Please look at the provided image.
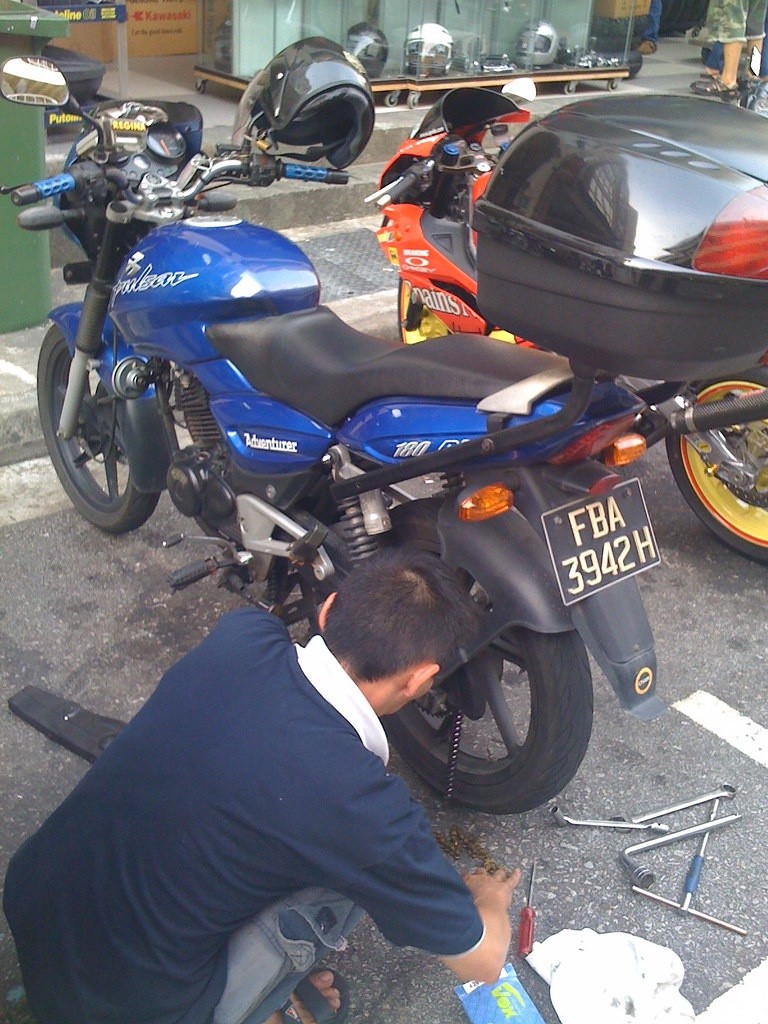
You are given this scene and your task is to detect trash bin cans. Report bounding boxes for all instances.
[0,0,74,338]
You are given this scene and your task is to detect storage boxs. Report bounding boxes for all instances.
[477,92,768,381]
[46,0,231,65]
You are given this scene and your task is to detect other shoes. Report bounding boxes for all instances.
[638,40,657,54]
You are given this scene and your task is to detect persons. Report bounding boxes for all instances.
[3,545,521,1024]
[689,0,768,100]
[635,0,662,55]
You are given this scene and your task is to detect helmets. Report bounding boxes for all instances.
[345,22,388,77]
[213,18,234,72]
[404,23,453,77]
[231,36,375,170]
[515,19,559,66]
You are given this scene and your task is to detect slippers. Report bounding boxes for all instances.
[700,72,721,80]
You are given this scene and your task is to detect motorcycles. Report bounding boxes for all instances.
[0,54,677,815]
[363,75,767,565]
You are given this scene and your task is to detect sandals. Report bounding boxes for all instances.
[689,77,738,104]
[736,77,748,90]
[279,968,348,1024]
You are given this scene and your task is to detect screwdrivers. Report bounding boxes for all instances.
[519,859,540,954]
